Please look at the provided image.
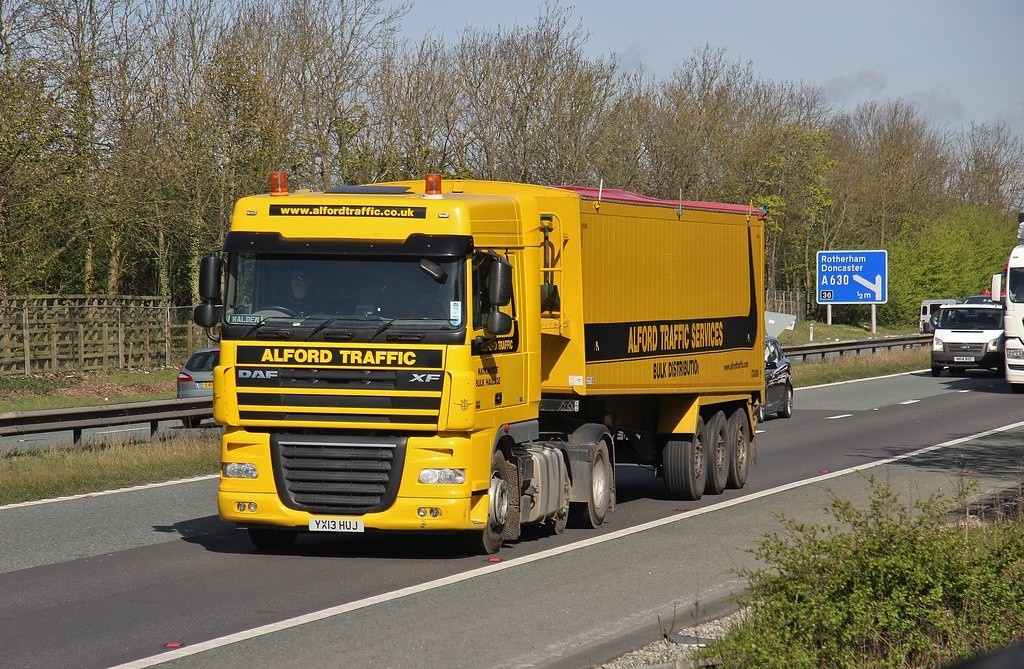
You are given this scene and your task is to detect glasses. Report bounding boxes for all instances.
[289,274,309,281]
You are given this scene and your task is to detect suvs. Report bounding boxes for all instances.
[919,300,962,334]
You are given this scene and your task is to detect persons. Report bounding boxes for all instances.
[375,268,447,318]
[270,266,326,317]
[978,313,987,320]
[953,311,961,323]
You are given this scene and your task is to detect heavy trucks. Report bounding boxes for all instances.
[193,172,766,555]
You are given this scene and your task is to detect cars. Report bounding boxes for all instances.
[754,336,793,423]
[177,348,220,427]
[931,304,1005,377]
[949,296,1005,320]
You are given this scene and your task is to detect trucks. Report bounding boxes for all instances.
[991,213,1024,393]
